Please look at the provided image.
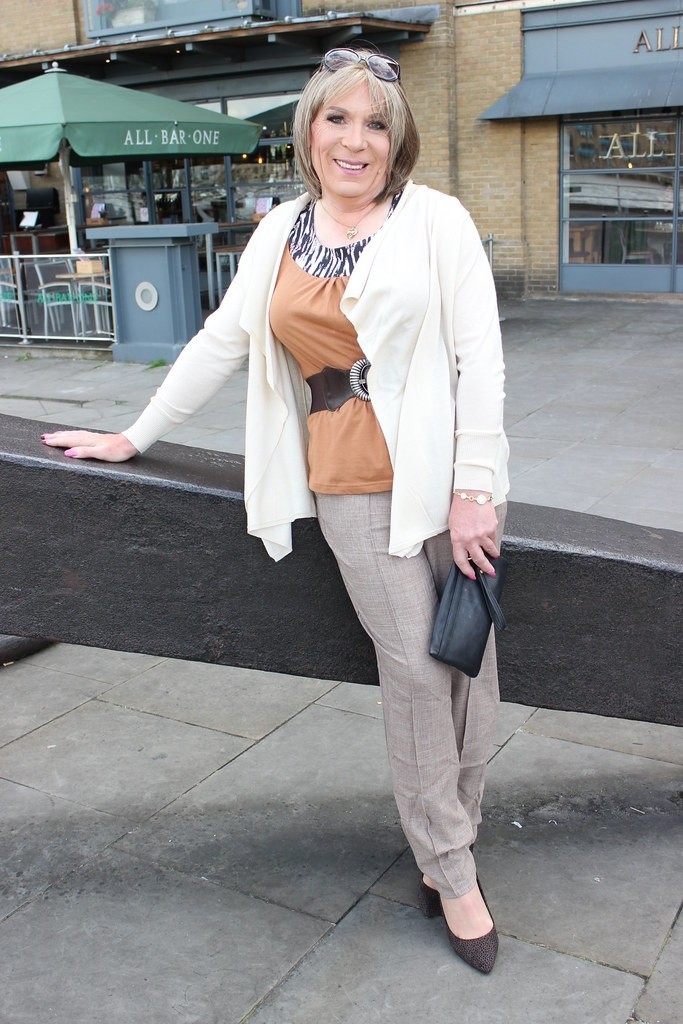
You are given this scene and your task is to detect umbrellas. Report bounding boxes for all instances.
[0,62,266,254]
[243,100,306,136]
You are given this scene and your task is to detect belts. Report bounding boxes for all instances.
[305,360,372,415]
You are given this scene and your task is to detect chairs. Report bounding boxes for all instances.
[618,228,655,264]
[1,256,115,343]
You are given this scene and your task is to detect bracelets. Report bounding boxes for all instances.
[450,490,494,504]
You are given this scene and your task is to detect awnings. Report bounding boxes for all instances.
[569,173,683,213]
[475,61,683,120]
[0,5,440,87]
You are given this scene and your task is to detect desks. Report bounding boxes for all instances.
[206,220,260,310]
[635,227,683,265]
[55,272,115,337]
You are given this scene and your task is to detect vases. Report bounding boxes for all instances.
[110,7,155,28]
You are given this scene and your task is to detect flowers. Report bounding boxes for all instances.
[96,0,158,20]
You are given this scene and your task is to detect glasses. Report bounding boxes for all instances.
[319,48,402,85]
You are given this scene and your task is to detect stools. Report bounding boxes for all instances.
[215,245,247,308]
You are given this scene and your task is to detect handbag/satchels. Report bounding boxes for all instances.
[429,542,509,678]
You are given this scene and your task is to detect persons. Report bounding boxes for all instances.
[40,48,510,976]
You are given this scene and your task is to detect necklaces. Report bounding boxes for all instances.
[317,197,380,238]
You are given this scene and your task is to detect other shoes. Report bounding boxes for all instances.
[440,866,498,974]
[416,841,474,918]
